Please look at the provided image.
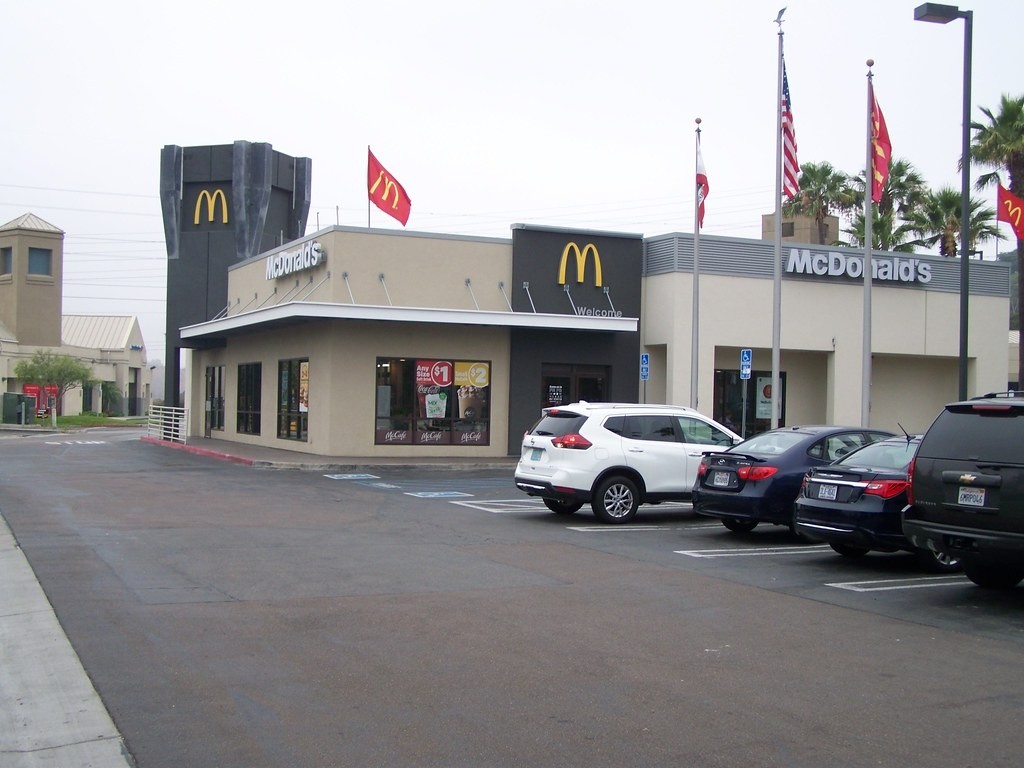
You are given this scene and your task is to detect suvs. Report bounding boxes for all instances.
[900,390,1024,592]
[514,400,782,523]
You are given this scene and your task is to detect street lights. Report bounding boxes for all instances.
[914,2,973,401]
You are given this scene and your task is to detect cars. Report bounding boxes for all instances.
[690,423,898,544]
[393,412,487,433]
[792,435,964,575]
